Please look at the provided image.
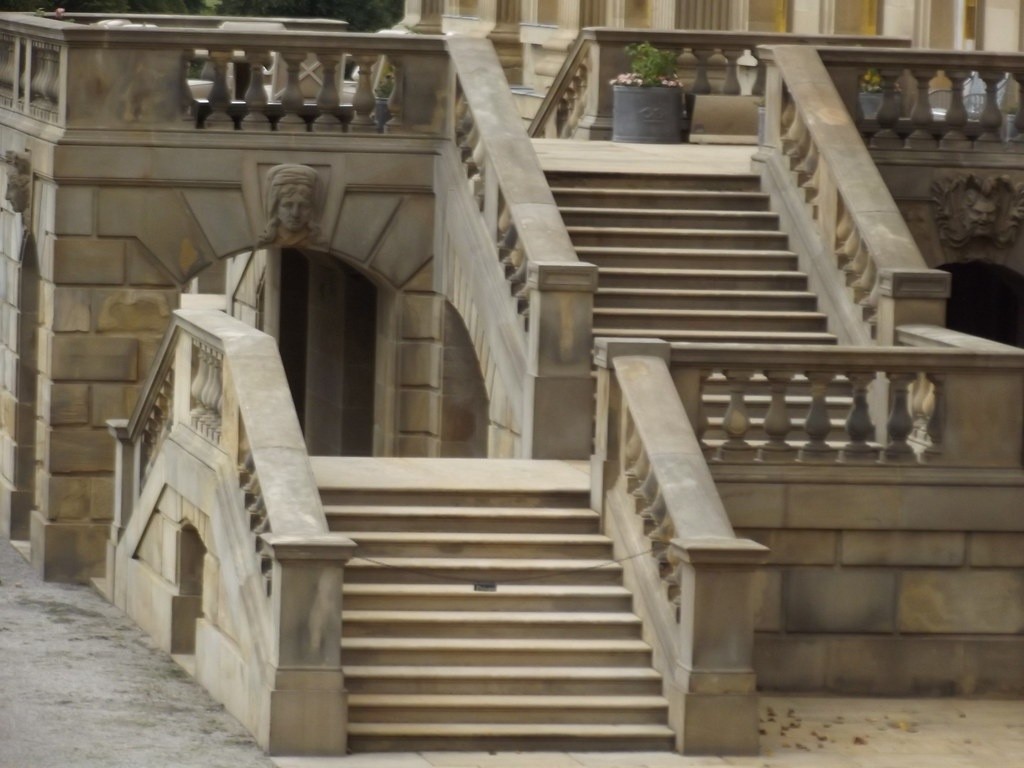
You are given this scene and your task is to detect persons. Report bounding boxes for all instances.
[925,171,1023,259]
[259,165,325,246]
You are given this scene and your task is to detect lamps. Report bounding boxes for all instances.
[331,104,357,132]
[296,104,323,132]
[191,98,214,129]
[260,102,287,132]
[226,100,250,130]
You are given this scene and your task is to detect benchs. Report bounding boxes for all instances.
[928,70,1010,123]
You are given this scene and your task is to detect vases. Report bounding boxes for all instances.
[859,93,904,121]
[610,84,684,145]
[373,98,395,133]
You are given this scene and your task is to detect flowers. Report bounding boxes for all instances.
[607,40,686,89]
[375,64,398,98]
[860,68,904,94]
